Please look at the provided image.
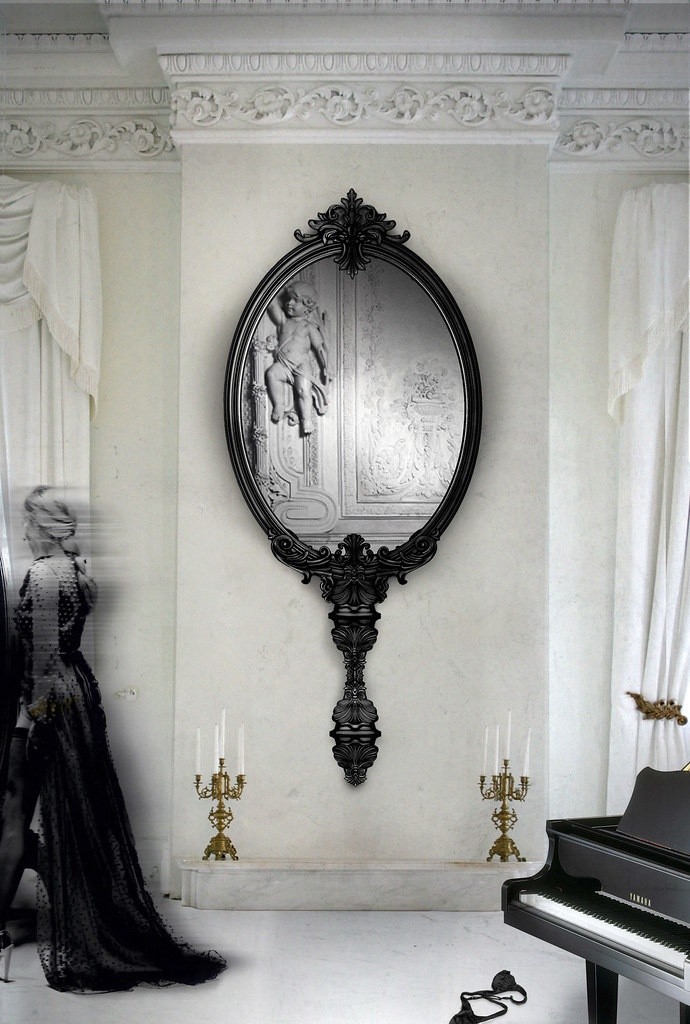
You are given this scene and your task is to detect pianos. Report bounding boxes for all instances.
[500,762,690,1024]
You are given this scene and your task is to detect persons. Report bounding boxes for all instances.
[264,279,333,434]
[1,485,229,994]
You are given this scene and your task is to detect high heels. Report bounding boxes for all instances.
[0,931,13,982]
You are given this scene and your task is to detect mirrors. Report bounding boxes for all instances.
[224,187,483,790]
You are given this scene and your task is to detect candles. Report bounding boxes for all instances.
[494,725,498,775]
[219,708,225,758]
[237,726,245,775]
[504,709,512,759]
[522,727,531,776]
[480,726,488,776]
[195,726,200,774]
[213,722,218,775]
